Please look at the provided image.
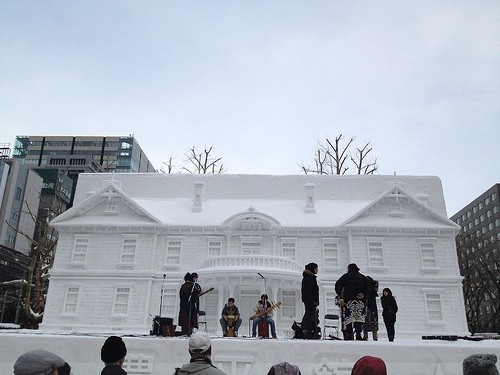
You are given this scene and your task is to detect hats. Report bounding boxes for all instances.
[351,356,387,375]
[101,336,126,363]
[267,362,301,375]
[463,354,499,375]
[189,332,211,354]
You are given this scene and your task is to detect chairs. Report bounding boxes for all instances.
[322,314,340,340]
[198,311,207,332]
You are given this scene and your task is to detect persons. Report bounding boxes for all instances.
[13,349,65,375]
[101,336,127,375]
[301,262,319,338]
[463,353,499,375]
[362,275,379,341]
[252,294,277,339]
[177,332,227,375]
[335,264,368,340]
[351,356,387,375]
[267,362,301,375]
[380,288,398,342]
[220,297,242,337]
[178,272,202,337]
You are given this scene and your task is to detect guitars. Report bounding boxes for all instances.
[198,288,214,297]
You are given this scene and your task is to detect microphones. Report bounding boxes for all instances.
[163,274,166,278]
[258,272,264,279]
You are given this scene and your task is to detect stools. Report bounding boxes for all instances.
[258,322,270,339]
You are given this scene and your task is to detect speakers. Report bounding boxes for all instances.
[153,317,173,335]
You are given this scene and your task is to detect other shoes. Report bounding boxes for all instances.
[272,333,277,338]
[223,330,227,336]
[252,333,256,337]
[356,339,362,341]
[362,338,368,341]
[374,339,377,341]
[304,333,315,339]
[389,340,394,342]
[233,329,236,337]
[346,338,354,340]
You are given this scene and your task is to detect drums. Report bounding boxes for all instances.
[226,315,236,337]
